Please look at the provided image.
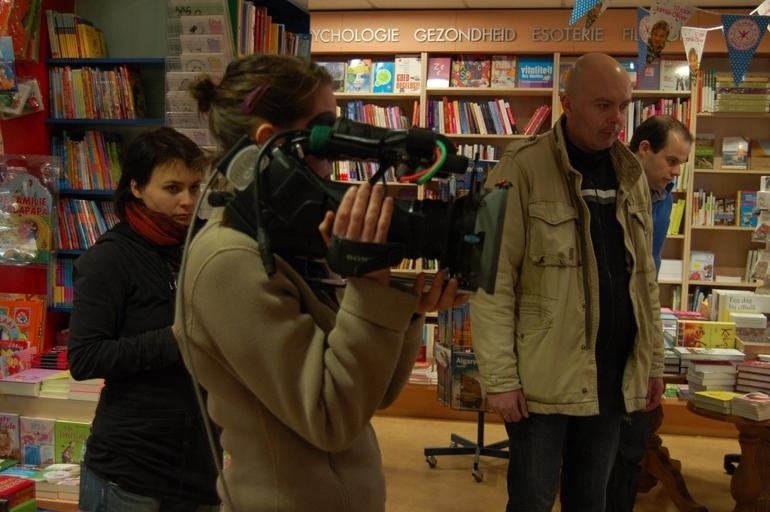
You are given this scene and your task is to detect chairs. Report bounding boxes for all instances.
[425,398,534,485]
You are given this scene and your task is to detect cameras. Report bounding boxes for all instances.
[206,116,514,297]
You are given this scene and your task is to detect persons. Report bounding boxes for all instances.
[172,54,469,511]
[468,54,666,511]
[68,126,221,512]
[606,114,694,510]
[386,257,442,271]
[649,18,670,51]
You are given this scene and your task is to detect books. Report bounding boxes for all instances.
[558,54,690,94]
[46,8,107,58]
[696,65,768,113]
[618,94,690,147]
[50,129,125,191]
[694,133,769,172]
[326,99,420,131]
[692,229,769,283]
[694,176,769,229]
[668,194,686,239]
[427,56,553,89]
[48,63,148,120]
[417,143,501,202]
[654,158,690,193]
[391,283,484,412]
[424,96,552,135]
[161,13,235,221]
[329,158,417,185]
[1,328,106,401]
[50,259,75,307]
[658,256,683,285]
[312,55,422,94]
[658,285,769,422]
[1,413,91,512]
[241,1,312,55]
[52,199,121,251]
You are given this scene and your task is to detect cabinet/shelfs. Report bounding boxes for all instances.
[312,9,770,440]
[47,58,165,376]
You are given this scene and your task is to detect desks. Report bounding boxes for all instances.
[686,401,769,512]
